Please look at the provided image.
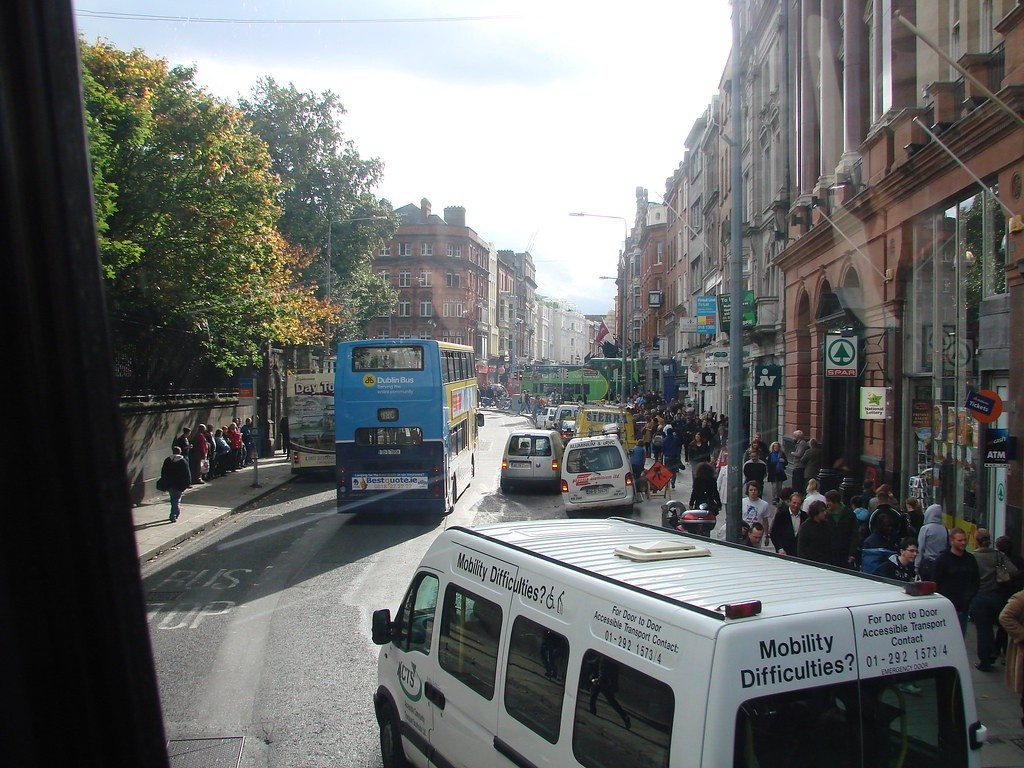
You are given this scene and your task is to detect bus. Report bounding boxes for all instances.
[333,339,485,522]
[286,366,336,474]
[520,356,647,408]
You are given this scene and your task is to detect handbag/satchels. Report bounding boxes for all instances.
[937,527,952,567]
[707,496,719,515]
[996,550,1010,581]
[642,427,646,435]
[905,513,916,536]
[775,452,784,473]
[156,477,167,491]
[200,458,209,473]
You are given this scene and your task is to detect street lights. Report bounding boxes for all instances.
[567,212,629,405]
[323,214,387,375]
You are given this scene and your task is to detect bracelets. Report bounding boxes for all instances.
[765,536,769,538]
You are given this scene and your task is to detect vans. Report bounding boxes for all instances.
[551,405,581,435]
[500,429,565,494]
[369,515,989,768]
[572,400,636,457]
[561,434,634,519]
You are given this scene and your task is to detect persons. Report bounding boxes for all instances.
[518,389,531,415]
[541,632,559,677]
[609,390,729,516]
[744,432,789,504]
[280,416,290,462]
[972,528,1019,670]
[771,460,981,692]
[477,385,501,406]
[999,590,1024,730]
[791,429,817,493]
[588,656,631,730]
[531,387,572,406]
[741,482,770,548]
[177,415,259,488]
[161,446,191,522]
[576,392,587,404]
[994,536,1024,664]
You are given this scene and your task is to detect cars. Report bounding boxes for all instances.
[561,419,577,447]
[534,407,558,430]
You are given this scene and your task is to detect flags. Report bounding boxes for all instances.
[596,321,609,342]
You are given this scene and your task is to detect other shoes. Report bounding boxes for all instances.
[990,657,995,663]
[975,663,991,671]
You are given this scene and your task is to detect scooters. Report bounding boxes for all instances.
[661,502,717,537]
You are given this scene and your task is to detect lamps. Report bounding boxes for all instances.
[904,144,925,155]
[963,96,986,110]
[790,214,816,226]
[812,196,823,207]
[931,122,952,135]
[775,231,796,241]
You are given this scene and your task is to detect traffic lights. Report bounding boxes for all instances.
[519,370,523,381]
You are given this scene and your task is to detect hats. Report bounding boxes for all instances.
[667,428,673,434]
[825,489,841,501]
